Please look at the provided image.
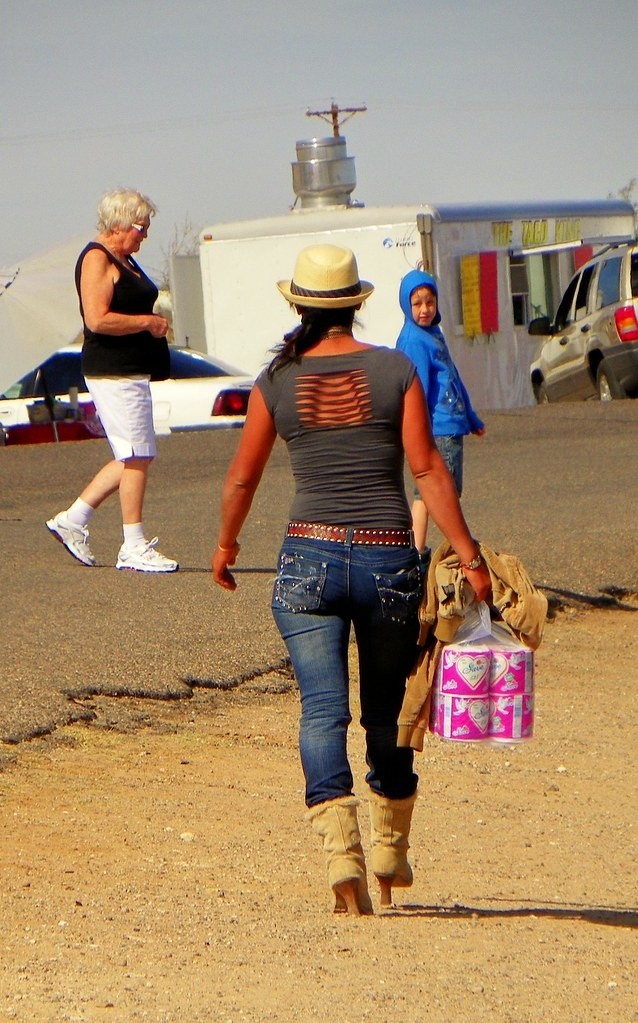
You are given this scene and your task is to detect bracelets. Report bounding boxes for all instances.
[463,556,481,569]
[218,542,238,552]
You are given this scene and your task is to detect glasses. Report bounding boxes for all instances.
[130,222,150,234]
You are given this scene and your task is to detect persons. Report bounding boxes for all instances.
[47,190,179,572]
[395,270,486,567]
[211,244,490,915]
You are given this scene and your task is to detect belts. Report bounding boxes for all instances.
[287,520,411,546]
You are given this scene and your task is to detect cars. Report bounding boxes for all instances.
[0,344,255,435]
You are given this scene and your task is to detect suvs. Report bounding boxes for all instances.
[527,240,638,403]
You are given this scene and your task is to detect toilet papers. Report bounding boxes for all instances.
[428,642,536,744]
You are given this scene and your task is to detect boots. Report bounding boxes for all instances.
[364,788,413,906]
[303,795,374,917]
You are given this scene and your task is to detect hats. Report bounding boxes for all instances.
[275,243,375,308]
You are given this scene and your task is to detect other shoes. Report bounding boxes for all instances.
[417,545,432,575]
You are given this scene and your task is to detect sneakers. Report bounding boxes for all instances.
[116,534,179,573]
[45,510,96,567]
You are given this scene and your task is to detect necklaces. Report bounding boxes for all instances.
[325,330,353,336]
